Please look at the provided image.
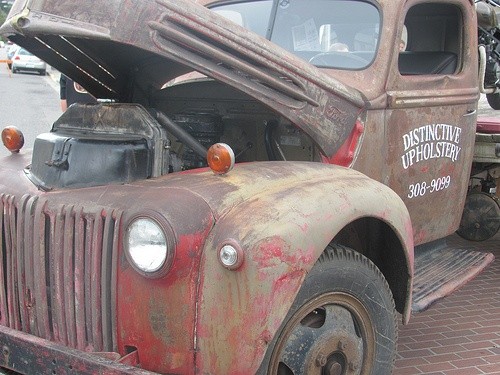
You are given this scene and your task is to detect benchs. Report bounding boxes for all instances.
[293,50,459,75]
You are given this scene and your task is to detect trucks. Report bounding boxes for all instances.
[0,0,500,375]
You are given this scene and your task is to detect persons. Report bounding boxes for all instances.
[60,74,95,113]
[330,43,348,51]
[400,40,405,51]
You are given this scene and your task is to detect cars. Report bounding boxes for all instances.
[8,44,46,75]
